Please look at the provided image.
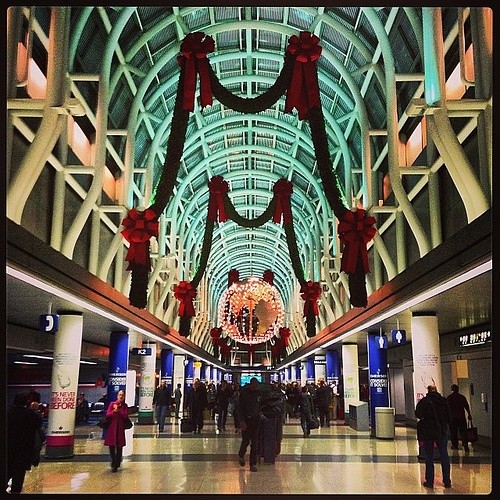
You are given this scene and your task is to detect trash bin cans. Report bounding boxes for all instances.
[375,406,396,441]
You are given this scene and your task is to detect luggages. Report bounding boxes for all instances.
[180,408,193,433]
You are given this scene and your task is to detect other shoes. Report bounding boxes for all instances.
[193,428,201,434]
[240,454,261,471]
[302,431,311,438]
[159,426,163,431]
[110,463,118,472]
[444,481,451,488]
[464,447,469,452]
[218,426,225,431]
[451,445,459,450]
[423,480,434,487]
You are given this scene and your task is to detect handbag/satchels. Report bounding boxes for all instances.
[464,420,478,442]
[97,418,109,428]
[308,415,319,429]
[124,417,133,429]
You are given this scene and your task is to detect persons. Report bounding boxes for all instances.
[315,380,331,427]
[329,381,340,420]
[7,394,41,493]
[75,392,88,425]
[236,378,259,473]
[184,378,317,434]
[103,390,128,473]
[447,384,472,452]
[174,383,182,419]
[415,385,452,489]
[150,381,170,432]
[296,387,314,438]
[98,394,107,406]
